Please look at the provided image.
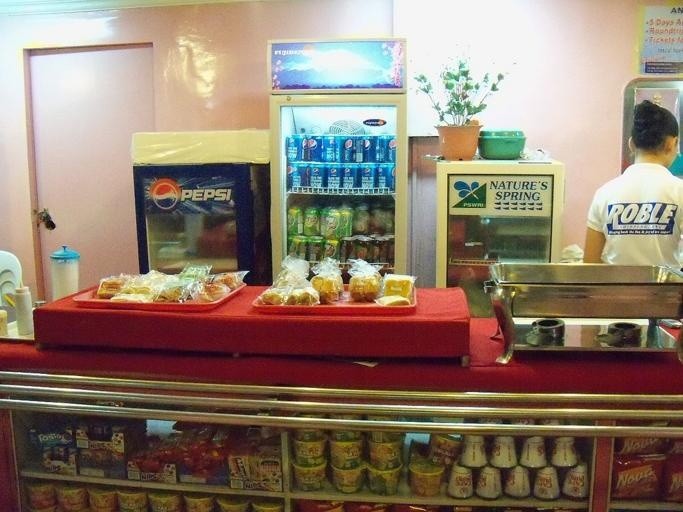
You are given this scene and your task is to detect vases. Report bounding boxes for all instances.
[409,135,440,156]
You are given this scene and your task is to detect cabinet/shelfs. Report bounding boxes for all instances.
[0,316,683,511]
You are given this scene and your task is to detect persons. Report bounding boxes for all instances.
[583,100,683,274]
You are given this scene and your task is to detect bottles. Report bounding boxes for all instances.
[14,281,34,335]
[0,311,8,334]
[52,245,81,300]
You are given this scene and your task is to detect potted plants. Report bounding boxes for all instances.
[412,42,517,161]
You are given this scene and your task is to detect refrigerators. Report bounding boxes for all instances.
[436,159,567,319]
[268,35,407,286]
[132,128,270,284]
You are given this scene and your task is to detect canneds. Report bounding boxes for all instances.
[286,162,396,194]
[286,133,396,162]
[286,199,396,237]
[285,238,396,269]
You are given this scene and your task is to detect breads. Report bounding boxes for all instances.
[349,275,381,302]
[378,294,417,306]
[98,272,239,305]
[383,278,414,298]
[259,274,341,307]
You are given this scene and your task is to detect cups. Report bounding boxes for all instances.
[447,435,589,500]
